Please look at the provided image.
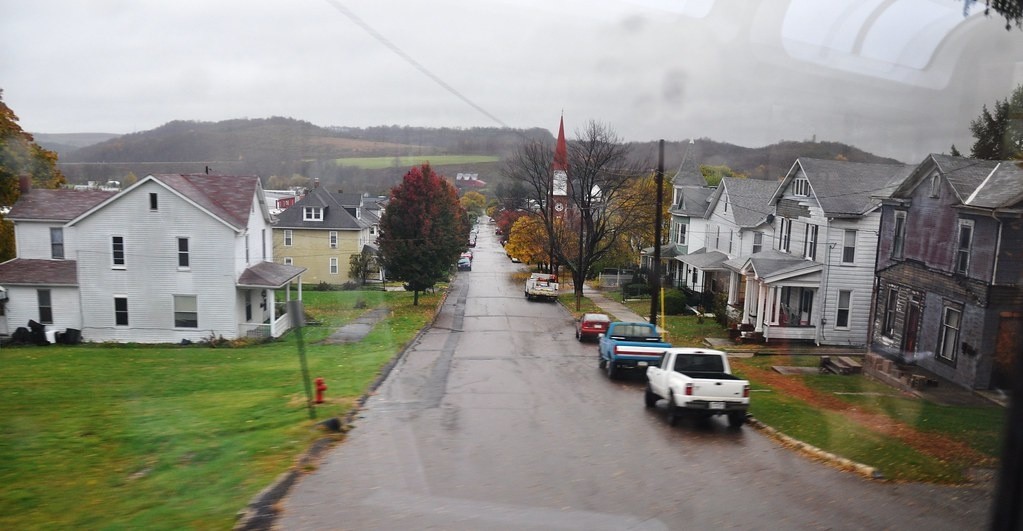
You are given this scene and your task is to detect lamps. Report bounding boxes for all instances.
[767,214,776,232]
[961,341,969,357]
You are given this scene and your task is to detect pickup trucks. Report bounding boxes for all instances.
[645,347,751,429]
[524,272,560,303]
[597,321,673,381]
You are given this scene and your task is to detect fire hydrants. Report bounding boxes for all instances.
[315,376,327,404]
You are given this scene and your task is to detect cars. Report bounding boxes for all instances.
[457,251,473,271]
[574,312,612,342]
[466,225,479,247]
[495,226,502,235]
[500,240,523,263]
